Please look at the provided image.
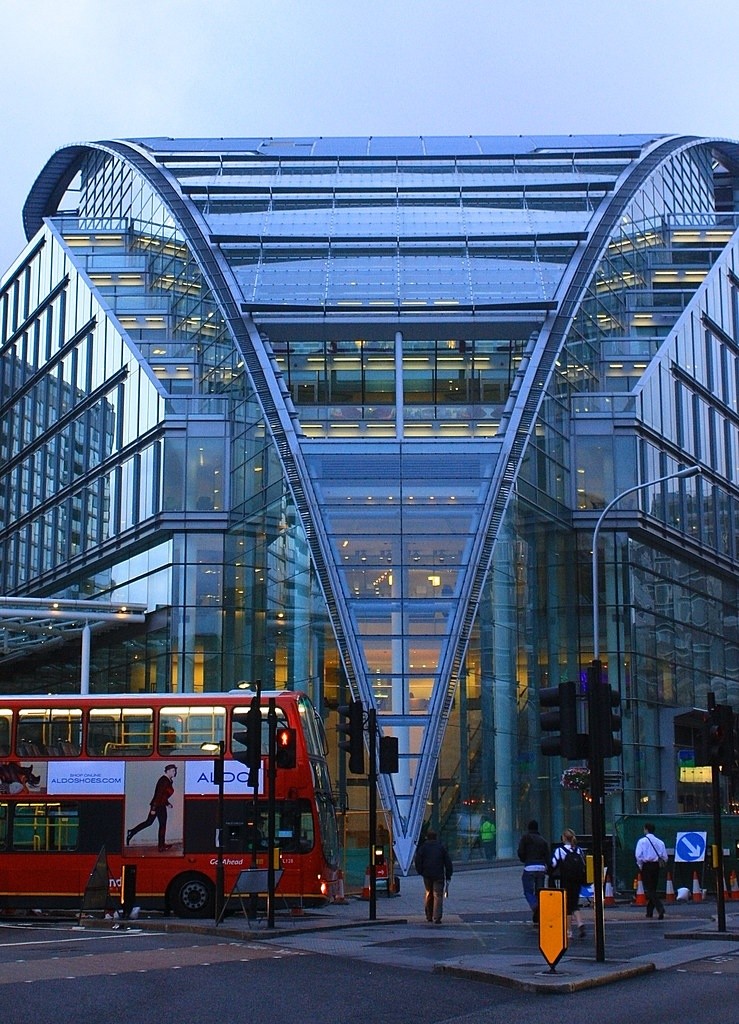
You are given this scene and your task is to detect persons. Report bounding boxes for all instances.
[160,726,176,749]
[126,764,178,852]
[415,832,453,924]
[479,816,496,857]
[551,829,587,940]
[244,816,273,851]
[517,820,554,930]
[377,824,390,836]
[634,822,669,919]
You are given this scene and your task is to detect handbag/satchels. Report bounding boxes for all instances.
[549,848,563,880]
[658,856,666,868]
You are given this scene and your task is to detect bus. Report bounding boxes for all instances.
[0,689,345,919]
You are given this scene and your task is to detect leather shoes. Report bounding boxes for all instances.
[159,845,172,852]
[126,830,132,846]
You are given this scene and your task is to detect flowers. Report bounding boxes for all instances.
[559,766,591,793]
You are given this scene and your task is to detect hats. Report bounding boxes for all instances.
[528,820,538,830]
[164,764,177,772]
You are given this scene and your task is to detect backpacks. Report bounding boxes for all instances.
[561,845,584,883]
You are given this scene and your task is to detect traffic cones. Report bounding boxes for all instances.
[730,870,739,902]
[723,876,730,902]
[603,876,619,908]
[631,874,650,906]
[663,872,681,905]
[686,871,709,904]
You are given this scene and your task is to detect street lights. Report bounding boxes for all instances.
[591,465,701,960]
[237,679,262,919]
[200,741,225,923]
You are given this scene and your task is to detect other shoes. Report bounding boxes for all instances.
[578,923,587,937]
[425,917,433,921]
[434,920,442,924]
[658,910,665,919]
[533,921,538,928]
[567,929,573,938]
[645,914,653,918]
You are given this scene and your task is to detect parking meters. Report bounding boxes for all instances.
[586,855,605,960]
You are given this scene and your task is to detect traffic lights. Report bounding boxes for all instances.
[336,703,364,754]
[600,682,623,757]
[234,709,261,769]
[539,681,575,757]
[709,723,739,766]
[277,728,296,768]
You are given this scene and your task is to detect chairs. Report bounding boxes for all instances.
[0,741,98,757]
[196,496,215,510]
